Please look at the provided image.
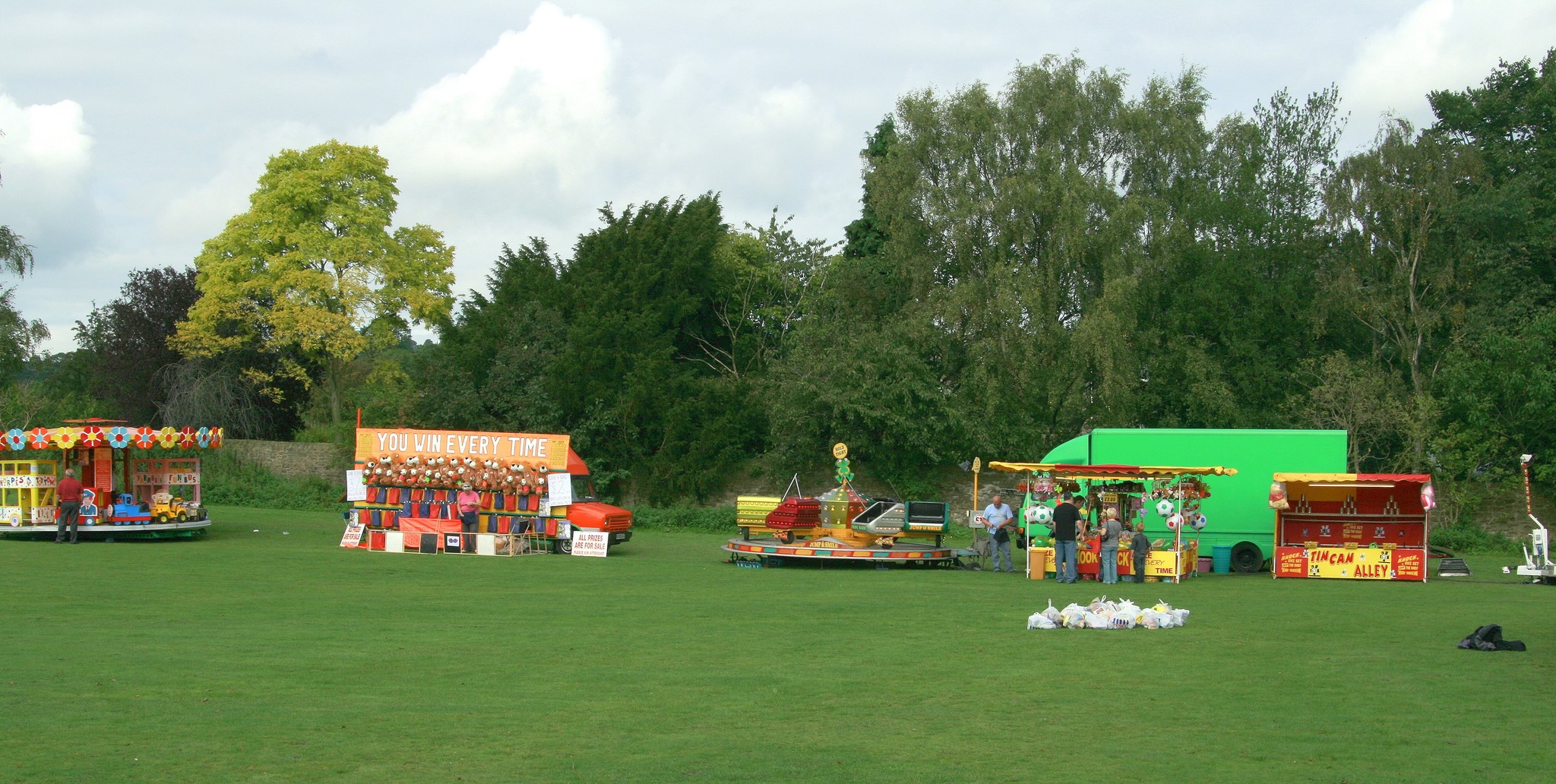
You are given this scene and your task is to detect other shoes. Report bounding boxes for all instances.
[1010,570,1016,573]
[996,570,1000,573]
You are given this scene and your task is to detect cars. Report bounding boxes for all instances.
[186,503,208,522]
[1470,461,1510,482]
[1410,458,1447,481]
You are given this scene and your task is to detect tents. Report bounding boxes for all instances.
[987,461,1237,584]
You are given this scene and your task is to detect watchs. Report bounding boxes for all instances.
[473,502,475,505]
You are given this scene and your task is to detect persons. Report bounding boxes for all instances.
[53,468,84,544]
[458,482,481,553]
[1053,492,1095,583]
[111,464,117,504]
[1097,508,1122,584]
[982,496,1018,573]
[1132,523,1151,583]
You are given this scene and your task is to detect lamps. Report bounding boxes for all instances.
[1309,483,1395,488]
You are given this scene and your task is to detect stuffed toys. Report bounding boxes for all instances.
[1269,483,1290,509]
[362,453,550,495]
[1421,483,1436,512]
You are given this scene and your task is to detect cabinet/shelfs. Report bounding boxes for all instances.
[352,484,566,519]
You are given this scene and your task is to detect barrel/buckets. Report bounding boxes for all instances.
[1212,545,1232,574]
[1067,611,1084,627]
[1198,558,1212,573]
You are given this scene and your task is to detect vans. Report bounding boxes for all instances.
[54,487,105,526]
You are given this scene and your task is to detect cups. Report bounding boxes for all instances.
[1295,494,1311,514]
[1383,495,1400,515]
[1340,495,1357,514]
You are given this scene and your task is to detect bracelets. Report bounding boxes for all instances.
[55,505,58,507]
[459,511,461,513]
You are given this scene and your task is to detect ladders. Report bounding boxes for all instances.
[1438,558,1472,577]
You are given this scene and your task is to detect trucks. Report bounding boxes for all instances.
[338,408,634,554]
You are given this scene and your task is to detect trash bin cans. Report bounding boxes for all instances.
[1029,550,1047,579]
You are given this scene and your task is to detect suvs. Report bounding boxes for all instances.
[150,497,187,524]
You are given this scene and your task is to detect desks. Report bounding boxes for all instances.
[1031,547,1101,582]
[398,517,467,550]
[1117,547,1198,583]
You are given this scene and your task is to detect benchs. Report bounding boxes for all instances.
[909,500,943,521]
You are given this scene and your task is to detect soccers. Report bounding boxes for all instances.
[1180,509,1194,525]
[1024,504,1055,530]
[1165,512,1185,531]
[1189,513,1207,532]
[1156,499,1175,517]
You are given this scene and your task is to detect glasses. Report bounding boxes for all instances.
[464,485,471,487]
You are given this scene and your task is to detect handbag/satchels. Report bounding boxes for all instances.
[461,511,476,525]
[1102,521,1109,541]
[1027,595,1189,630]
[349,487,571,539]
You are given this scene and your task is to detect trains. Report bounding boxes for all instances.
[107,491,152,525]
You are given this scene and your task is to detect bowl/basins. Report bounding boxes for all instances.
[1079,623,1084,628]
